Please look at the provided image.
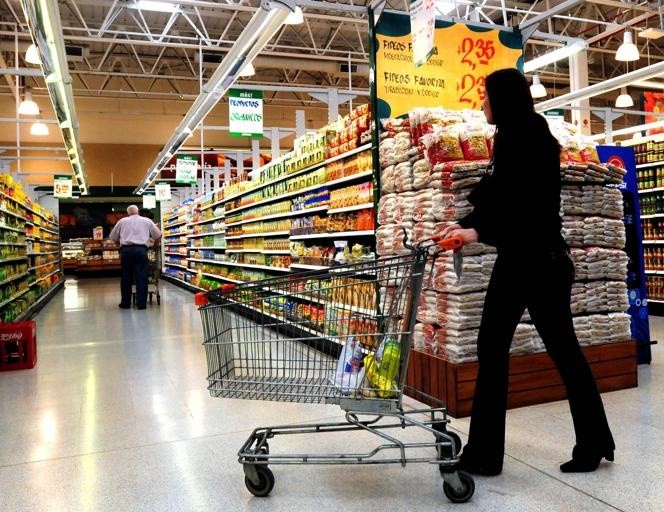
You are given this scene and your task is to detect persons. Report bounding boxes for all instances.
[109,204,162,310]
[440,67,616,477]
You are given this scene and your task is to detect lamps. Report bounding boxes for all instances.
[18,89,40,115]
[523,38,589,74]
[31,111,49,135]
[615,86,634,108]
[529,74,548,98]
[615,25,640,62]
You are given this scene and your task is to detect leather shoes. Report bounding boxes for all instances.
[118,304,146,310]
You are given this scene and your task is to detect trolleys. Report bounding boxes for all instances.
[193,232,475,505]
[129,246,161,306]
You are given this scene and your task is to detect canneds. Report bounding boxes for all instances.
[633,140,664,189]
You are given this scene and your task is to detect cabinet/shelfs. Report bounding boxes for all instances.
[0,191,122,322]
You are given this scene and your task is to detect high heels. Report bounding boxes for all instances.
[458,456,501,475]
[560,451,614,472]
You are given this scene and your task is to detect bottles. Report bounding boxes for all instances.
[634,140,664,302]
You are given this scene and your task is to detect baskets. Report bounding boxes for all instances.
[0,321,37,371]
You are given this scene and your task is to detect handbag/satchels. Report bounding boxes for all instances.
[466,174,520,222]
